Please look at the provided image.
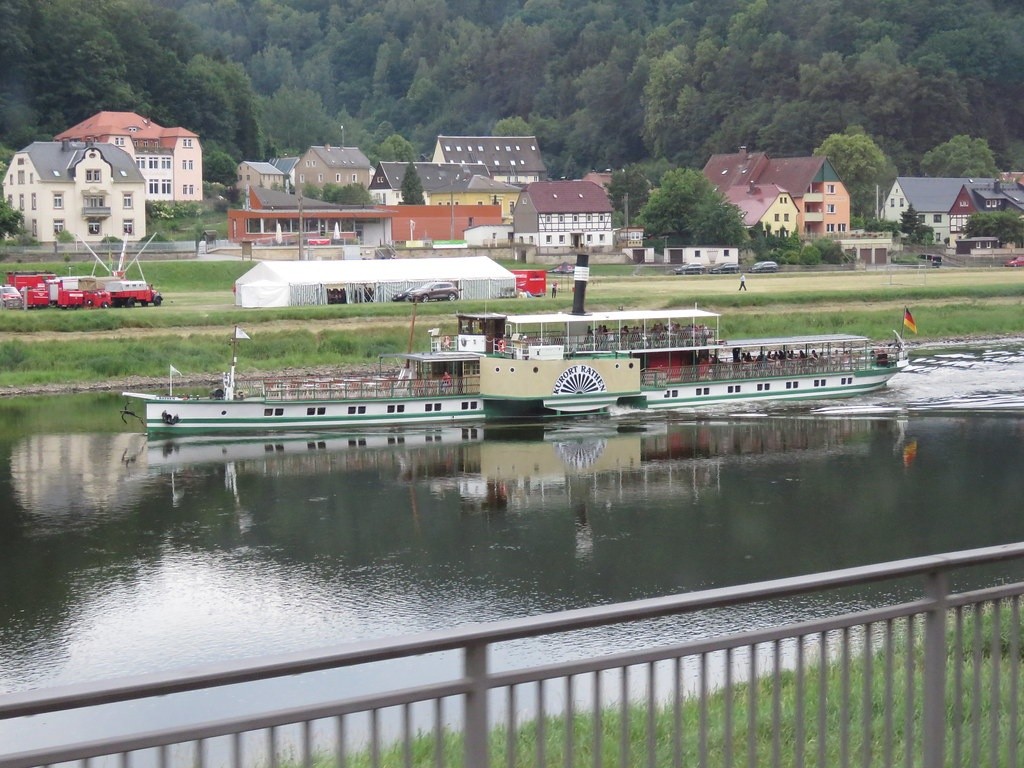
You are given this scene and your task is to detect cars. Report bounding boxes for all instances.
[392,286,418,303]
[1006,256,1024,267]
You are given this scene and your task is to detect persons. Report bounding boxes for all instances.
[326,287,374,304]
[551,282,558,298]
[586,322,710,348]
[150,283,153,290]
[440,371,452,395]
[738,273,747,292]
[694,349,875,378]
[232,283,236,299]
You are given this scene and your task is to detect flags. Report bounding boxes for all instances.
[235,327,251,340]
[903,305,917,334]
[169,364,183,378]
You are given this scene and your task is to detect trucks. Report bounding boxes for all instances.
[7,270,164,309]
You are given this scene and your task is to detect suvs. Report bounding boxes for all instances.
[708,262,741,274]
[0,285,24,310]
[407,282,461,302]
[917,254,942,268]
[673,262,706,275]
[748,262,779,274]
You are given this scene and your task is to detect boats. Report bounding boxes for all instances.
[145,385,906,526]
[121,253,911,448]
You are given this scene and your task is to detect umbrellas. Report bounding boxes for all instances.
[276,223,283,245]
[332,222,341,240]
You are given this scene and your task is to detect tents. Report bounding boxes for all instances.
[547,262,575,292]
[234,254,516,309]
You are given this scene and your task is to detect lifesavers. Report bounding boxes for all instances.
[443,336,451,346]
[498,340,506,353]
